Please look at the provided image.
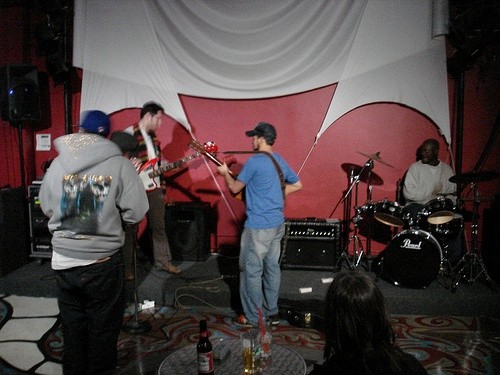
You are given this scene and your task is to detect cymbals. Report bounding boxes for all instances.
[448,169,495,184]
[340,162,384,186]
[359,151,396,168]
[459,208,481,222]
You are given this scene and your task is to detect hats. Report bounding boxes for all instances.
[71,111,110,136]
[246,123,276,138]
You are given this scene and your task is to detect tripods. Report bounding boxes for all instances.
[449,180,494,293]
[334,159,374,274]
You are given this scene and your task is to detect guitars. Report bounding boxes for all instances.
[137,141,219,193]
[186,138,246,201]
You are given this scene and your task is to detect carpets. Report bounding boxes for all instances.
[0,294,500,375]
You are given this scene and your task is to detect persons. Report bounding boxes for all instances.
[217,123,303,327]
[38,109,150,375]
[307,272,430,375]
[111,101,182,317]
[402,138,457,207]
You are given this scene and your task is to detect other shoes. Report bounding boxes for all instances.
[233,315,270,327]
[111,367,124,375]
[125,272,136,281]
[265,315,281,325]
[156,262,183,274]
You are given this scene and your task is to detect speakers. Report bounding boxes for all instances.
[279,218,343,271]
[0,186,34,276]
[0,64,42,122]
[163,200,211,262]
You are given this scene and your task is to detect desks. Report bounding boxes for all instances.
[158,339,307,375]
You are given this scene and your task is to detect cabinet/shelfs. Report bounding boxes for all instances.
[28,185,53,264]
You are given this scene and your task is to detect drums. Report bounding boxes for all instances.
[374,198,463,291]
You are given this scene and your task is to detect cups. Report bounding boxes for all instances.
[243,334,257,374]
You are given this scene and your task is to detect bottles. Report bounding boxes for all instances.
[197,319,214,375]
[256,316,272,369]
